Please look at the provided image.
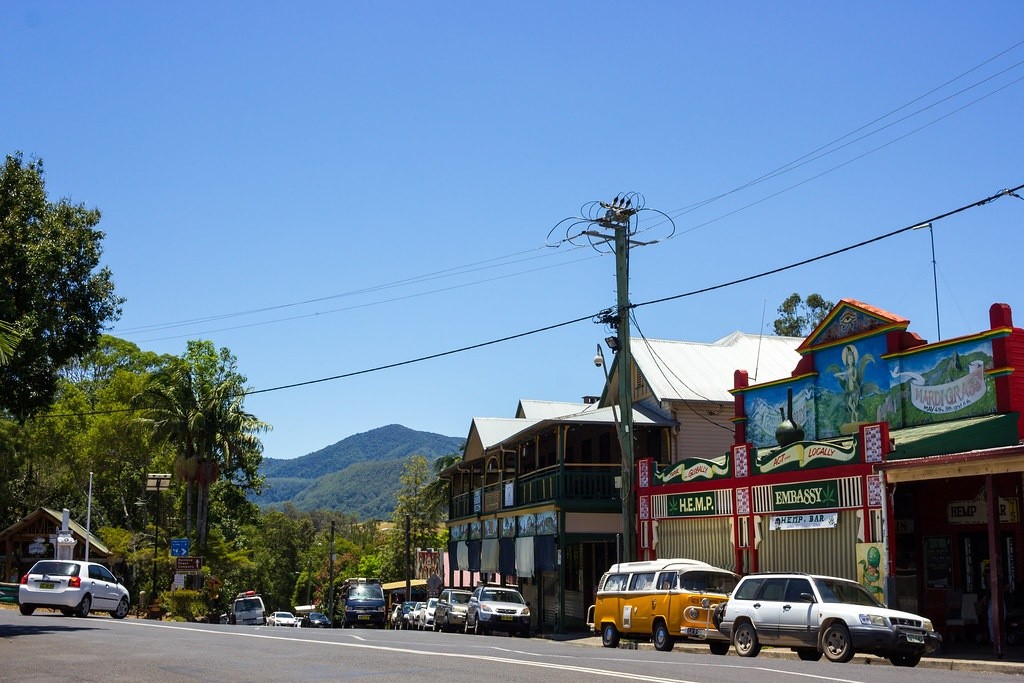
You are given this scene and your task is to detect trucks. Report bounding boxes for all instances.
[340,578,389,629]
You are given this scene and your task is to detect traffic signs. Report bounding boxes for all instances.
[175,557,203,571]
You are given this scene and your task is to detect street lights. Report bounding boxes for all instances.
[912,222,940,341]
[592,343,633,562]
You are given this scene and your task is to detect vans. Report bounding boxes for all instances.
[232,596,269,626]
[586,558,744,655]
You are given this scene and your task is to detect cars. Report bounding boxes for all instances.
[719,571,939,668]
[295,612,332,629]
[390,598,446,631]
[19,559,131,618]
[432,588,474,634]
[464,585,531,638]
[268,611,297,627]
